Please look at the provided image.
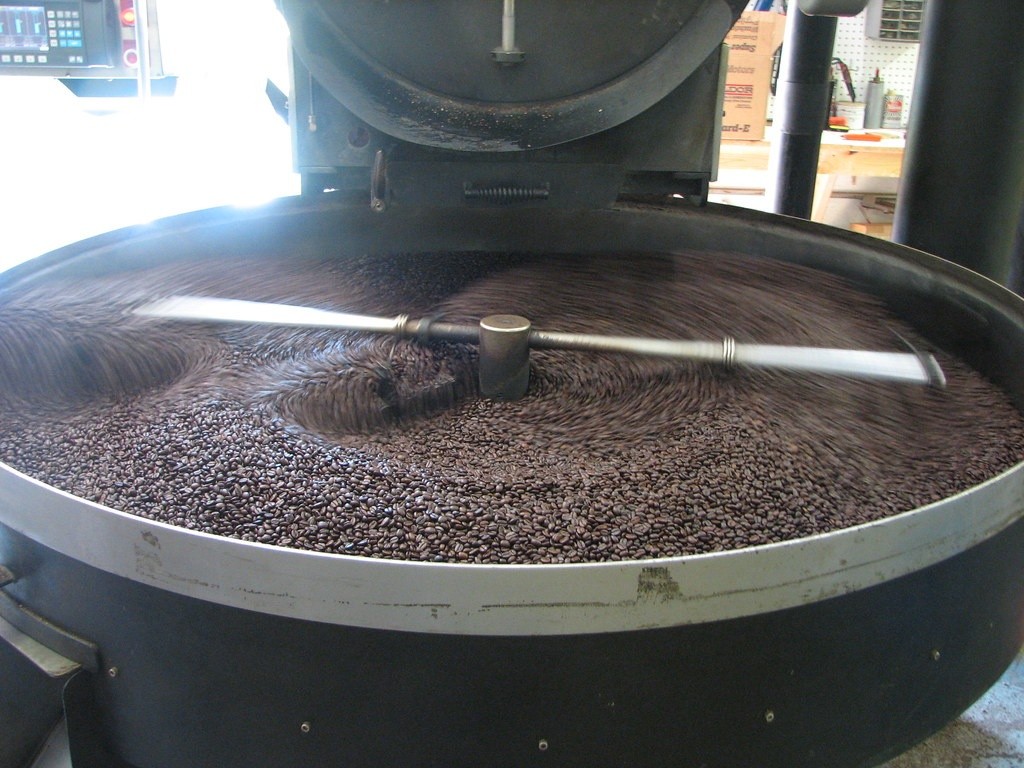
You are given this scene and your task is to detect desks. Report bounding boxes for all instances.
[708,126,905,225]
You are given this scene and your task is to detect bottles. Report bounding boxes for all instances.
[863,69,884,129]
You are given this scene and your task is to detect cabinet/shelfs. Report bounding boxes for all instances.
[866,0,923,43]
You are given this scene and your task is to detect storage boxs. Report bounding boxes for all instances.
[724,10,786,140]
